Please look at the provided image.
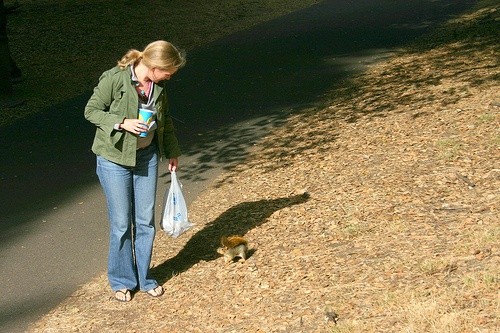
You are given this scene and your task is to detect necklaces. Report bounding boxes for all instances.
[137,82,149,96]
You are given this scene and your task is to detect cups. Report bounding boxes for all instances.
[137,108,154,137]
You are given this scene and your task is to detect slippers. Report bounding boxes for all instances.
[146,285,165,297]
[115,289,132,302]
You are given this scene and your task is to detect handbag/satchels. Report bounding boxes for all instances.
[159,169,191,237]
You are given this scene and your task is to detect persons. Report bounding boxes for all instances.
[85,41,185,302]
